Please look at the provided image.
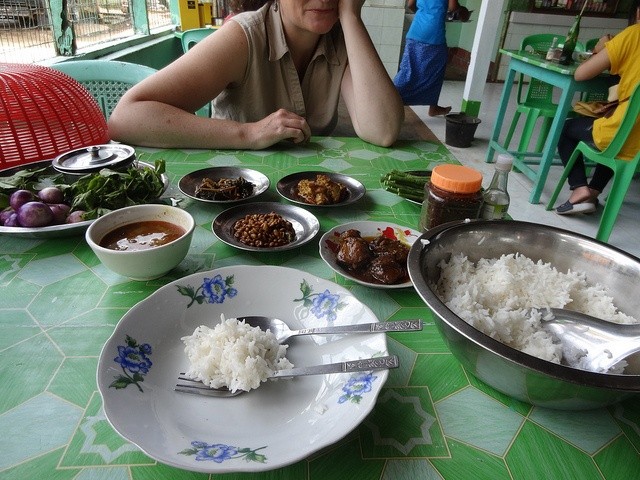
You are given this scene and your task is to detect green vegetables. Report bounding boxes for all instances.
[64,151,165,220]
[0,164,64,191]
[0,193,9,211]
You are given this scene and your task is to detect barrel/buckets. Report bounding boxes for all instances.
[444,112,482,147]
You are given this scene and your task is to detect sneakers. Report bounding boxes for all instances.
[429,103,453,117]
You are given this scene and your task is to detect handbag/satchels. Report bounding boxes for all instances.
[574,99,618,118]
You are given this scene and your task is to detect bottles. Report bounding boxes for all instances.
[476,153,514,219]
[558,15,581,66]
[545,37,559,60]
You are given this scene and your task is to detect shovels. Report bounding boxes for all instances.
[526,302,640,379]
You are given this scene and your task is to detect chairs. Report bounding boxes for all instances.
[45,60,159,124]
[181,28,219,119]
[503,33,585,173]
[546,83,640,243]
[582,38,621,102]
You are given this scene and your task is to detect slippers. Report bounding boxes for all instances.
[555,199,595,214]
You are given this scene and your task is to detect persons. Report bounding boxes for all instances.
[106,0,405,152]
[394,0,468,116]
[556,5,640,215]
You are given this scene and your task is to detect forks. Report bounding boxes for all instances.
[174,354,400,399]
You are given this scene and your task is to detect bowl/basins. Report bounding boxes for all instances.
[406,219,640,414]
[52,152,137,185]
[84,203,195,282]
[580,51,593,60]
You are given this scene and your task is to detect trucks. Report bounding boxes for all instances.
[1,0,99,32]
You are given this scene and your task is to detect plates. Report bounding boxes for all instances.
[275,170,366,208]
[319,220,430,290]
[0,158,169,243]
[178,166,271,203]
[398,170,432,206]
[211,201,320,253]
[96,264,388,474]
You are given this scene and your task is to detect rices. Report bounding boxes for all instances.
[181,313,294,393]
[314,401,327,415]
[437,249,639,374]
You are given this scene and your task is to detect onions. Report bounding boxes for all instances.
[48,204,70,224]
[0,205,16,226]
[4,212,19,227]
[65,210,86,224]
[17,202,53,227]
[38,187,63,204]
[10,190,34,212]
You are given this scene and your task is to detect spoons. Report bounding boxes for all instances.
[521,307,640,374]
[237,316,425,344]
